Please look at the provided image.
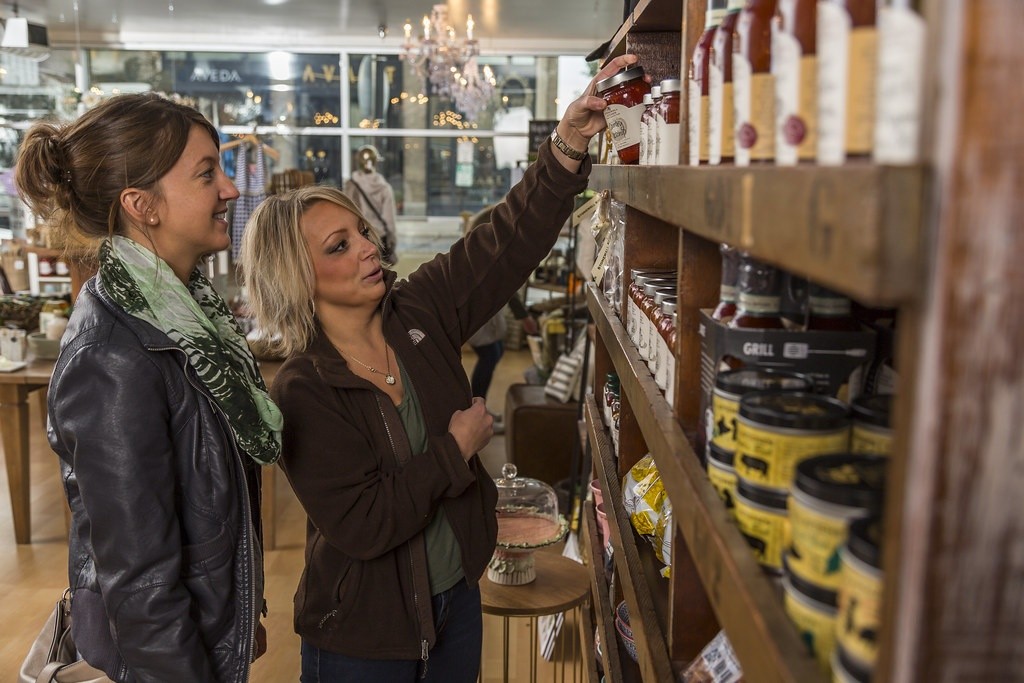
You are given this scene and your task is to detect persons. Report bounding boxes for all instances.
[468,291,538,436]
[16,93,283,683]
[241,54,654,683]
[345,145,395,262]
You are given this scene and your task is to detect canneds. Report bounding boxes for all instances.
[607,241,896,683]
[594,0,938,165]
[39,257,71,277]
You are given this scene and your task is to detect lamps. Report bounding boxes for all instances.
[430,49,499,120]
[398,10,479,77]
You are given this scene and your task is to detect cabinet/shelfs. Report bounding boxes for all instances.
[23,246,94,305]
[517,1,1014,683]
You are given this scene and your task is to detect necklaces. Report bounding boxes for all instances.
[334,338,396,384]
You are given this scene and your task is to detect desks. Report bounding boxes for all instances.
[479,553,590,683]
[0,350,287,552]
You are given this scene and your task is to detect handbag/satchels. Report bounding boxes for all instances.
[19,588,116,683]
[385,231,396,251]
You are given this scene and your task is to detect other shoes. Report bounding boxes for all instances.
[491,421,506,432]
[485,409,503,422]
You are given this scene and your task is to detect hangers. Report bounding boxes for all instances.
[217,122,281,160]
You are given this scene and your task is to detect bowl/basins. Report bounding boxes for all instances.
[615,619,637,663]
[617,597,635,638]
[26,331,59,359]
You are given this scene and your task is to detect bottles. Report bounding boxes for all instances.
[546,0,1024,683]
[45,311,68,342]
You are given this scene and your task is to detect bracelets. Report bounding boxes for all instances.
[462,455,471,469]
[550,128,588,161]
